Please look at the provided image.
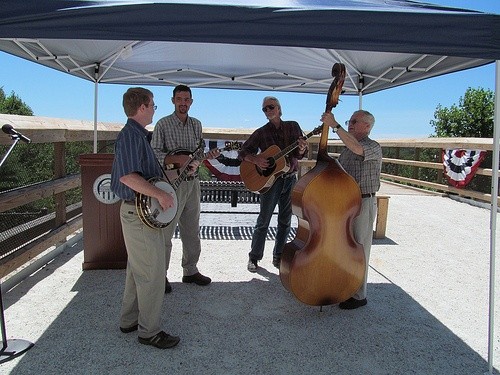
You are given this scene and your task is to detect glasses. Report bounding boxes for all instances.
[345,120,356,126]
[262,104,277,112]
[154,106,157,110]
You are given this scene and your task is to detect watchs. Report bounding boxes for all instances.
[333,124,341,132]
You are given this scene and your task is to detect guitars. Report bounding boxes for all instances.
[164,140,245,183]
[135,137,207,230]
[239,123,325,195]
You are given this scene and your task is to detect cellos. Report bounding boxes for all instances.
[279,62,366,313]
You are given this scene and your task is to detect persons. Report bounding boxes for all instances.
[320,111,383,310]
[238,97,309,272]
[150,85,220,294]
[111,87,180,347]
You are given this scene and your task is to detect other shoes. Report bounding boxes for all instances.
[273,262,280,268]
[247,259,259,272]
[165,277,171,293]
[339,297,367,309]
[182,273,211,285]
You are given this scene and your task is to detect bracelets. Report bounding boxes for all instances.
[299,148,305,151]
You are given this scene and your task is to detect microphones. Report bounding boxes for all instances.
[2,124,32,144]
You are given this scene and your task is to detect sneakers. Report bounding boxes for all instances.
[120,324,138,333]
[138,330,180,348]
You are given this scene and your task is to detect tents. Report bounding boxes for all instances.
[0,0,500,375]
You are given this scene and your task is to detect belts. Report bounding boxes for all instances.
[175,175,198,181]
[362,193,375,198]
[281,172,295,179]
[124,200,136,205]
